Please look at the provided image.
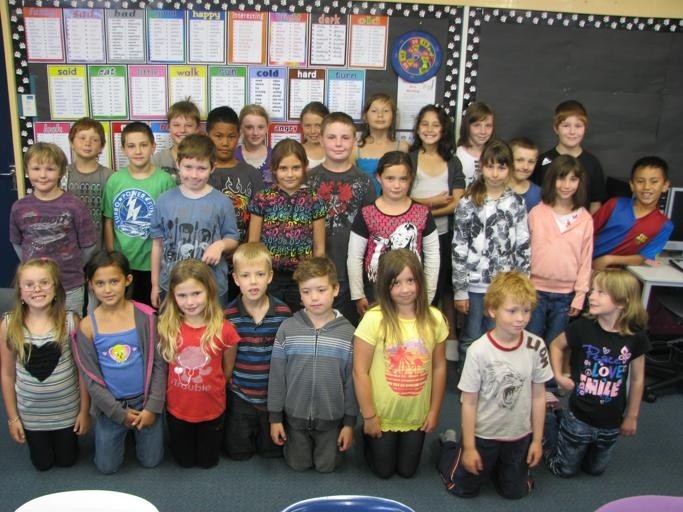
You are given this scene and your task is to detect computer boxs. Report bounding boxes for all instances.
[604,176,668,212]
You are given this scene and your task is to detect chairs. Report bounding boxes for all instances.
[282,492,413,511]
[10,488,161,512]
[595,492,681,511]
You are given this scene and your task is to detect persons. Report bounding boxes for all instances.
[8,90,675,407]
[269,254,359,475]
[155,258,243,471]
[349,247,450,481]
[540,267,654,480]
[0,255,91,475]
[221,240,293,464]
[74,247,169,477]
[433,270,553,501]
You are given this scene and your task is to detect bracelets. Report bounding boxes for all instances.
[5,416,22,427]
[624,413,640,420]
[360,413,377,421]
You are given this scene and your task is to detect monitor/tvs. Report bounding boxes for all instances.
[663,187,683,251]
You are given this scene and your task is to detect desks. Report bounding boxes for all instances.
[627,249,683,324]
